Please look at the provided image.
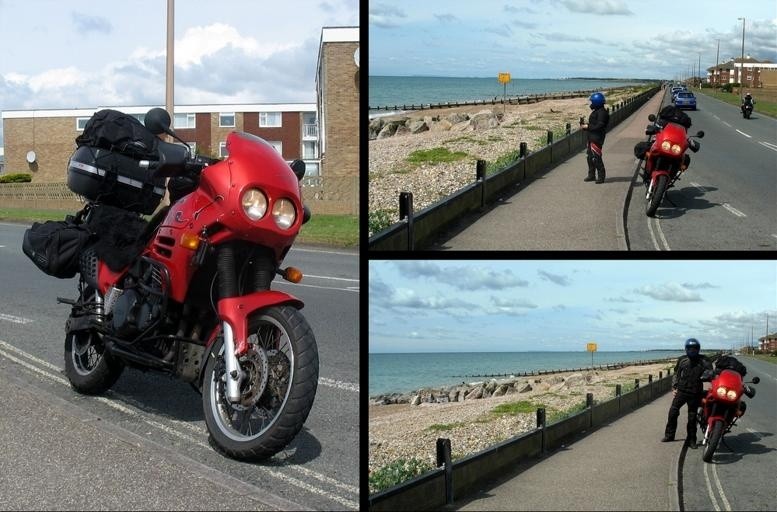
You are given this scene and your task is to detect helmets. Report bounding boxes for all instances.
[590,93,605,111]
[685,338,701,357]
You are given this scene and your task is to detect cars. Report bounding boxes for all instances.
[668,82,696,111]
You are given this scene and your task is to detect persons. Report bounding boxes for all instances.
[741,93,753,114]
[581,93,609,183]
[661,339,713,449]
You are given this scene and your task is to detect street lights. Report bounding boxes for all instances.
[678,54,702,90]
[737,17,746,97]
[711,36,721,98]
[731,311,768,357]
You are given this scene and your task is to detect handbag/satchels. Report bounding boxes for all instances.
[659,105,692,128]
[711,355,747,377]
[75,108,161,162]
[634,125,658,160]
[21,212,93,280]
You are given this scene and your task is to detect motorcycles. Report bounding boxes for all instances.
[738,96,756,119]
[55,132,319,464]
[697,353,759,463]
[633,105,704,219]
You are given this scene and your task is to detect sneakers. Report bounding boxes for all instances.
[595,177,604,184]
[584,176,596,181]
[662,436,676,442]
[686,436,698,450]
